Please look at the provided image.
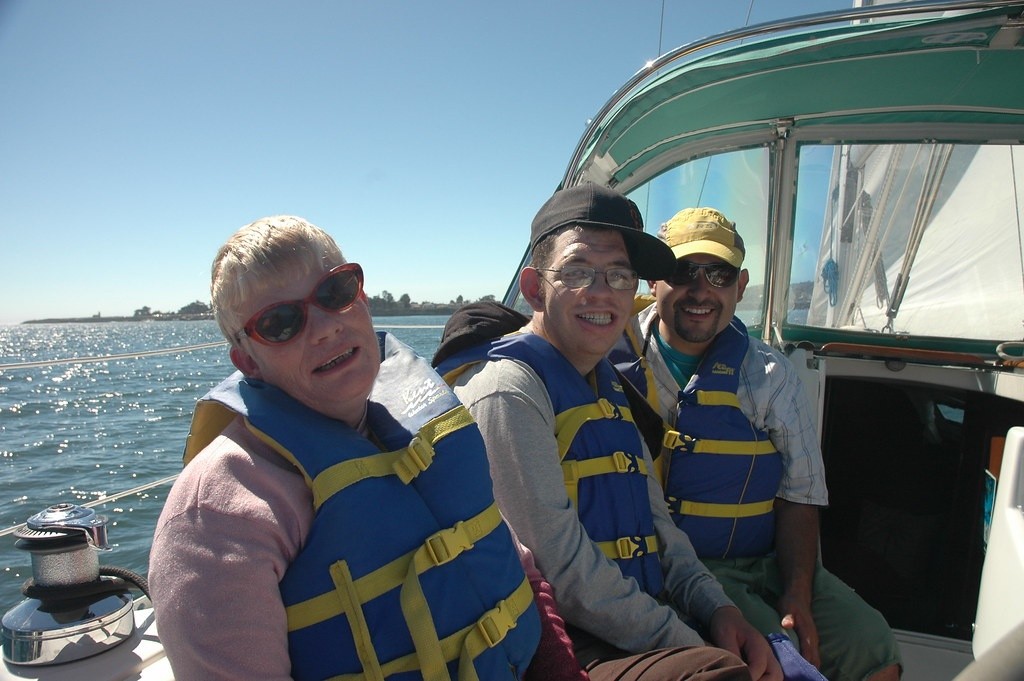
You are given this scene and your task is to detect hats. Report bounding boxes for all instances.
[530,183,676,280]
[655,206,746,268]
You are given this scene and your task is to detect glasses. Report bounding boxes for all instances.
[535,264,639,290]
[232,262,364,347]
[667,260,741,288]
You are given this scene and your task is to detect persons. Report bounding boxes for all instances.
[432,206,904,681]
[147,216,587,681]
[433,180,784,681]
